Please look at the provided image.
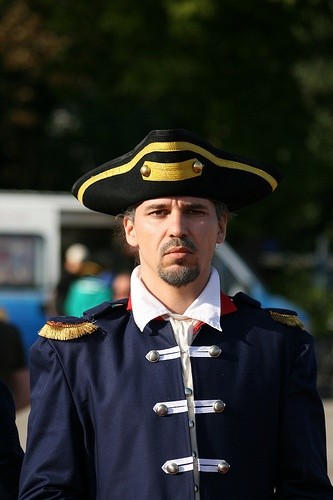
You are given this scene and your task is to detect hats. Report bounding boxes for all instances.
[71,127,290,221]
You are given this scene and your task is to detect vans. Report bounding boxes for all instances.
[0,190,312,358]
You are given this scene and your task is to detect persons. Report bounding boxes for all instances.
[0,237,133,500]
[19,131,332,500]
